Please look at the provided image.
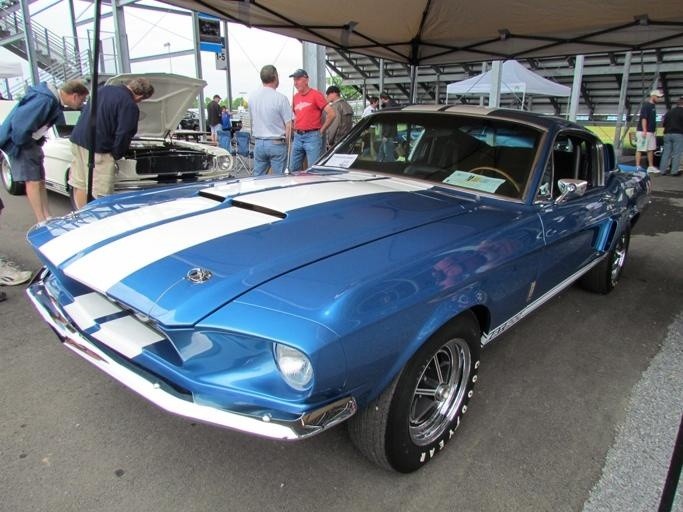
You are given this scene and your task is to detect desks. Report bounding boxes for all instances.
[173,129,212,143]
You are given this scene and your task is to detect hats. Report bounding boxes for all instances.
[289,69,307,77]
[650,90,664,97]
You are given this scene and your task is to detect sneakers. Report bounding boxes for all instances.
[0,258,32,286]
[646,167,660,173]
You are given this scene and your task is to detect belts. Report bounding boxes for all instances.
[297,129,318,134]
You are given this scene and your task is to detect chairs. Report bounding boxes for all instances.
[217,130,239,171]
[230,131,254,177]
[402,124,477,181]
[475,146,552,199]
[553,150,597,200]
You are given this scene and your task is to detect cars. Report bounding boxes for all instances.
[176,109,242,139]
[29,103,652,475]
[1,72,235,212]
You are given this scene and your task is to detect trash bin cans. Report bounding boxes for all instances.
[235,131,250,156]
[217,130,231,150]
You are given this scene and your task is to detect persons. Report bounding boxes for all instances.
[360,91,398,162]
[69,76,154,211]
[206,95,232,153]
[289,67,336,174]
[247,64,295,178]
[324,85,354,153]
[635,89,664,174]
[0,80,89,224]
[659,97,683,176]
